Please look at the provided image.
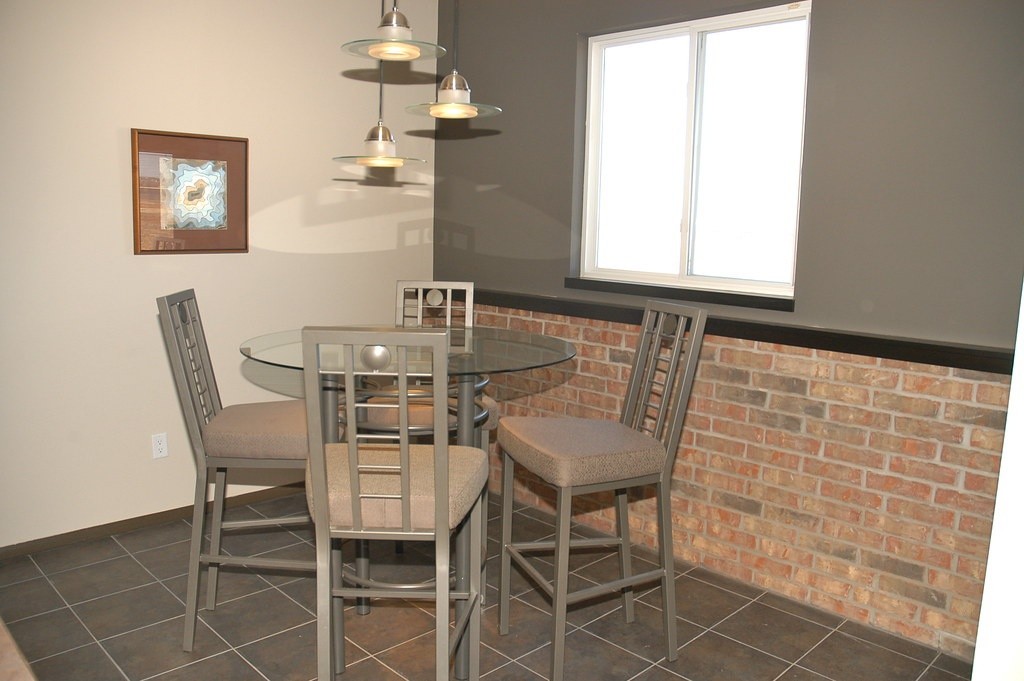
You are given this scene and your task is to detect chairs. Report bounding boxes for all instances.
[156,287,344,654]
[499,299,709,681]
[366,280,475,554]
[300,326,489,681]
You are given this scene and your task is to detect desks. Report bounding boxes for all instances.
[241,324,575,615]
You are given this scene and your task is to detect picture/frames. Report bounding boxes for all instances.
[132,127,249,256]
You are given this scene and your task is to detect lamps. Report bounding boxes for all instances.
[405,0,503,120]
[332,0,427,168]
[340,0,448,62]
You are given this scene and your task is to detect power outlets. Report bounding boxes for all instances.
[151,433,169,459]
